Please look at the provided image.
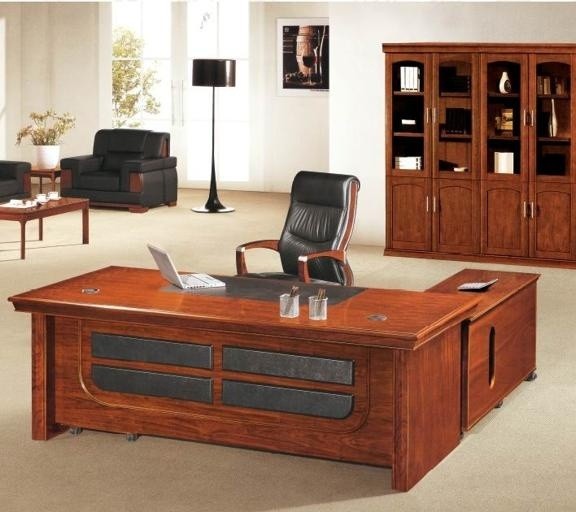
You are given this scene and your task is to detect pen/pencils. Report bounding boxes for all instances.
[282,284,298,315]
[313,288,326,314]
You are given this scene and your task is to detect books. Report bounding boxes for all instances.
[393,167,422,171]
[400,118,416,124]
[465,74,470,92]
[394,156,423,162]
[442,127,470,134]
[393,162,424,167]
[399,66,420,93]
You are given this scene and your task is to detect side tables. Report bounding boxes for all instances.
[30,168,60,194]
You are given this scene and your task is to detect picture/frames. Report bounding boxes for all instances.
[276,17,329,98]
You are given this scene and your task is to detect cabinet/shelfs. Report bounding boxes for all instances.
[382,43,576,270]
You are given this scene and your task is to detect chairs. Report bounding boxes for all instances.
[236,170,360,287]
[60,128,178,213]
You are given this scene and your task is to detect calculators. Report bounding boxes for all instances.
[457,278,499,291]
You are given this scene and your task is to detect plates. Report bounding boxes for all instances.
[35,199,50,203]
[47,197,61,200]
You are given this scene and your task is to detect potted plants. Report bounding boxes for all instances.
[14,107,77,169]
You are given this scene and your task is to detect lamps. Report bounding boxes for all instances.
[191,59,236,214]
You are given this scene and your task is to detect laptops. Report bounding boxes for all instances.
[147,241,226,289]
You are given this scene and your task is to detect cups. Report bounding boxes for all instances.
[308,294,329,320]
[279,293,300,319]
[36,193,47,201]
[47,191,58,198]
